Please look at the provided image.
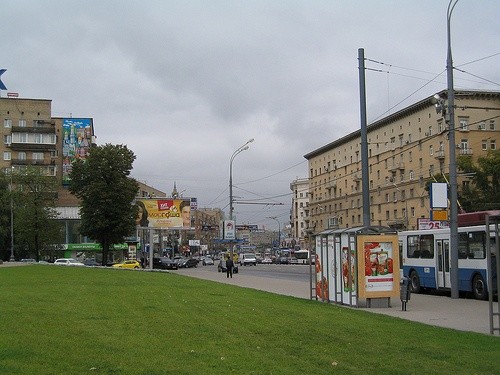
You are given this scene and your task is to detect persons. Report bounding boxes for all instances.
[82,252,86,263]
[174,245,190,257]
[163,251,168,257]
[226,256,234,278]
[140,256,145,268]
[273,238,296,249]
[134,201,149,227]
[177,201,191,227]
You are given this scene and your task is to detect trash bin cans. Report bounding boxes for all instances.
[400,278,411,300]
[233,265,238,273]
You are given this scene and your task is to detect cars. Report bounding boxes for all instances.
[218,260,239,273]
[173,248,239,269]
[83,261,101,266]
[54,258,85,267]
[21,259,49,264]
[112,260,143,270]
[255,248,318,268]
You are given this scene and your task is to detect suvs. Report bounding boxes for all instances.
[239,254,257,266]
[153,257,179,270]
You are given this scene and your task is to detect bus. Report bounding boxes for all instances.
[398,176,500,301]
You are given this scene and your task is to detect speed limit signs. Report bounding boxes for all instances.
[417,218,441,230]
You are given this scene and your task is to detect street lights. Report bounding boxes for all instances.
[230,138,256,261]
